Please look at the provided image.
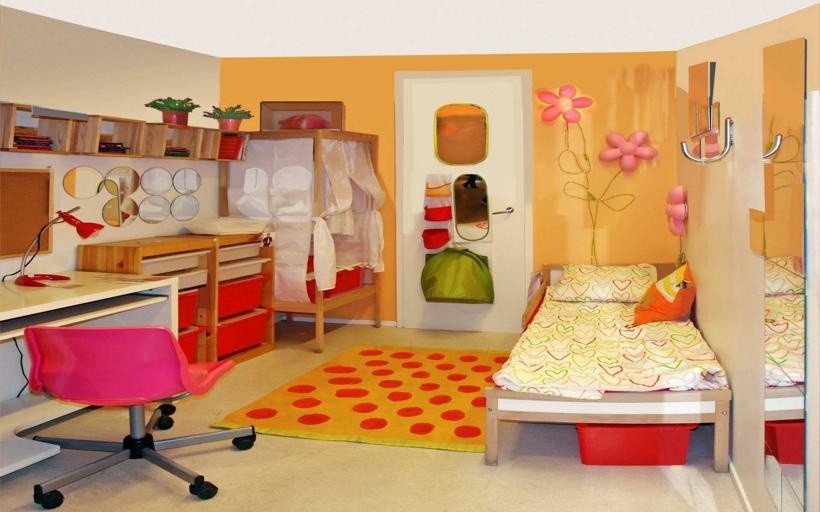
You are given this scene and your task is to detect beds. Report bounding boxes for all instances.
[765,255,807,422]
[483,261,730,471]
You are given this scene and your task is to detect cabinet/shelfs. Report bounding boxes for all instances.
[78,236,278,363]
[218,132,380,354]
[1,103,220,161]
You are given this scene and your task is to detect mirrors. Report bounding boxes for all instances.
[62,165,202,227]
[761,37,809,506]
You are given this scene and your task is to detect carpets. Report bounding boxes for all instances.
[209,346,509,450]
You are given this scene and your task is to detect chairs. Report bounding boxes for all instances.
[26,320,256,512]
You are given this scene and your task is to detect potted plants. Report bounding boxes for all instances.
[202,103,251,130]
[144,99,197,125]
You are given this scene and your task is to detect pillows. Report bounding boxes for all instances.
[635,264,695,326]
[551,263,656,305]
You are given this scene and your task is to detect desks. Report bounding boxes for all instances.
[0,274,181,495]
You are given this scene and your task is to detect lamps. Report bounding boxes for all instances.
[14,204,104,288]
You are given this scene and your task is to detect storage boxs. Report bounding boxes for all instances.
[209,311,271,352]
[199,277,266,318]
[764,424,805,466]
[172,327,200,359]
[305,268,367,297]
[177,290,201,324]
[576,423,696,468]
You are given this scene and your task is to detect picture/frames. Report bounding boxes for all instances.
[258,99,345,133]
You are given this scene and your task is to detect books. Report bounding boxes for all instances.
[13,133,54,151]
[98,141,130,153]
[165,146,190,158]
[218,133,250,160]
